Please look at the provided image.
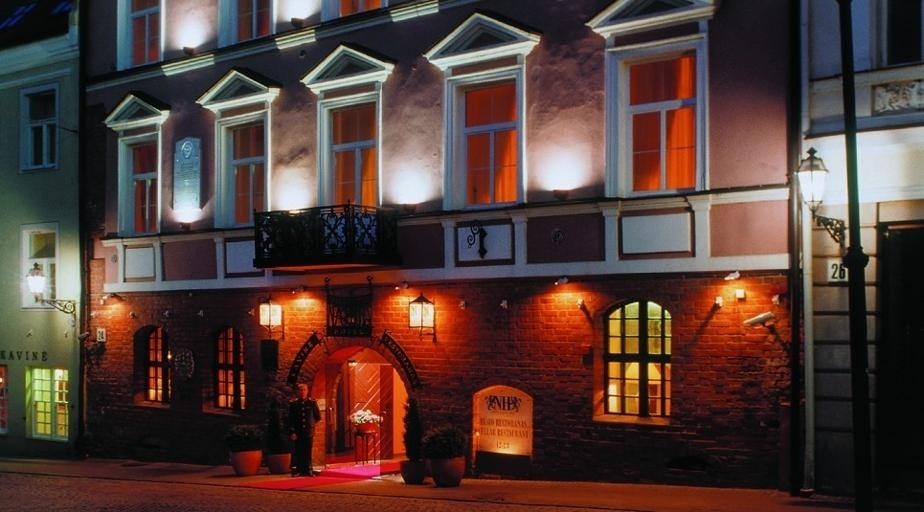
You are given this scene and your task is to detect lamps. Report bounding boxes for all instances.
[395,282,408,290]
[179,221,191,232]
[407,293,436,343]
[292,286,304,293]
[403,204,417,215]
[552,190,570,201]
[723,271,740,280]
[100,293,123,307]
[26,262,76,320]
[291,16,305,28]
[625,360,662,412]
[794,147,845,258]
[554,277,567,285]
[259,294,284,341]
[182,46,195,56]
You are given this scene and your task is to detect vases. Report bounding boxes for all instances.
[355,423,376,432]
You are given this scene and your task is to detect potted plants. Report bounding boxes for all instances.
[427,427,467,487]
[399,399,424,484]
[267,414,290,475]
[226,429,263,475]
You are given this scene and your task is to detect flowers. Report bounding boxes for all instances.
[350,409,382,424]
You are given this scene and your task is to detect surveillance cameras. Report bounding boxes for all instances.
[77,332,90,343]
[742,311,774,327]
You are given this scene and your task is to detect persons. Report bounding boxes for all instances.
[288,383,321,477]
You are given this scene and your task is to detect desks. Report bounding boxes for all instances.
[354,431,376,466]
